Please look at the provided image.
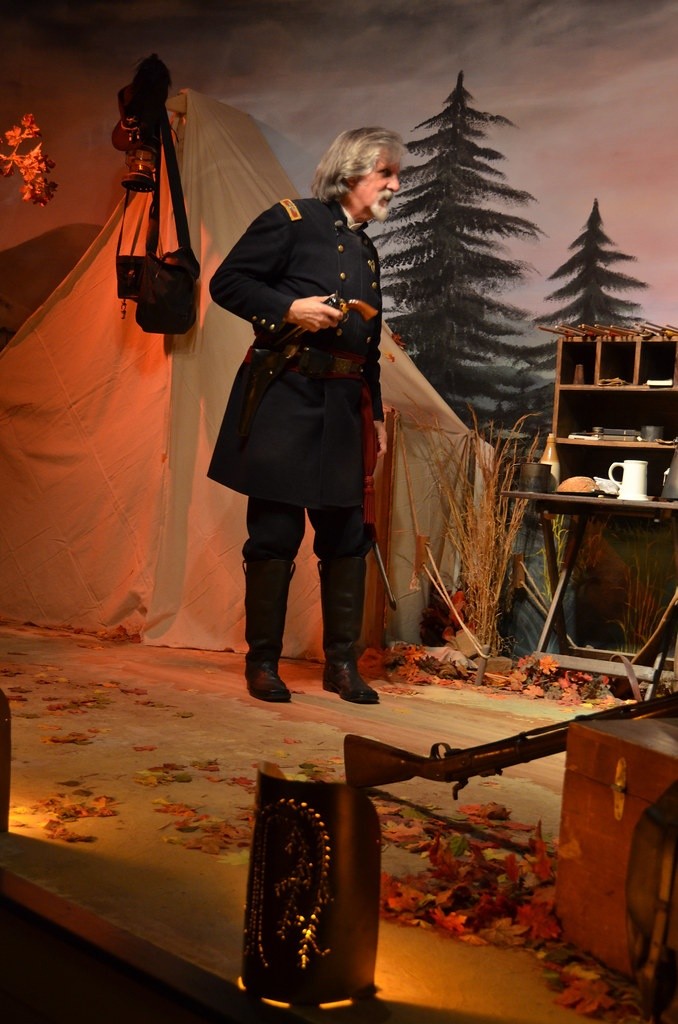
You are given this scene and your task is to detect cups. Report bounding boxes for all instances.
[520,463,551,492]
[608,459,648,500]
[641,426,664,442]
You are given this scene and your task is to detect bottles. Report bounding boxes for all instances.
[540,432,559,493]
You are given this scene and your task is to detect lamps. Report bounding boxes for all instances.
[122,134,162,191]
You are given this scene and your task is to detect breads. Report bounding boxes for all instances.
[556,476,599,492]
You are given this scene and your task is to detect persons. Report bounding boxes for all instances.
[206,125,405,705]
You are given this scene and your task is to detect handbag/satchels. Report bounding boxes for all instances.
[136,250,200,335]
[116,255,145,300]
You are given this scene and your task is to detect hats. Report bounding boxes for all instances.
[111,58,168,151]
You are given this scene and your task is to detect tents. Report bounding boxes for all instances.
[1,89,551,660]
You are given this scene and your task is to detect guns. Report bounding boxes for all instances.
[343,693,678,789]
[275,290,377,340]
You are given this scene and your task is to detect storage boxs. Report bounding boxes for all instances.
[551,715,678,980]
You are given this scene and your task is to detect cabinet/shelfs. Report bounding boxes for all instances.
[550,334,678,495]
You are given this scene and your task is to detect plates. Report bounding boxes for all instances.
[548,490,619,498]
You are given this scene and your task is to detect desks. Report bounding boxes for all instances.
[499,490,678,681]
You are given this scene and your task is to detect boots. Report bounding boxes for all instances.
[317,556,379,704]
[241,559,291,701]
